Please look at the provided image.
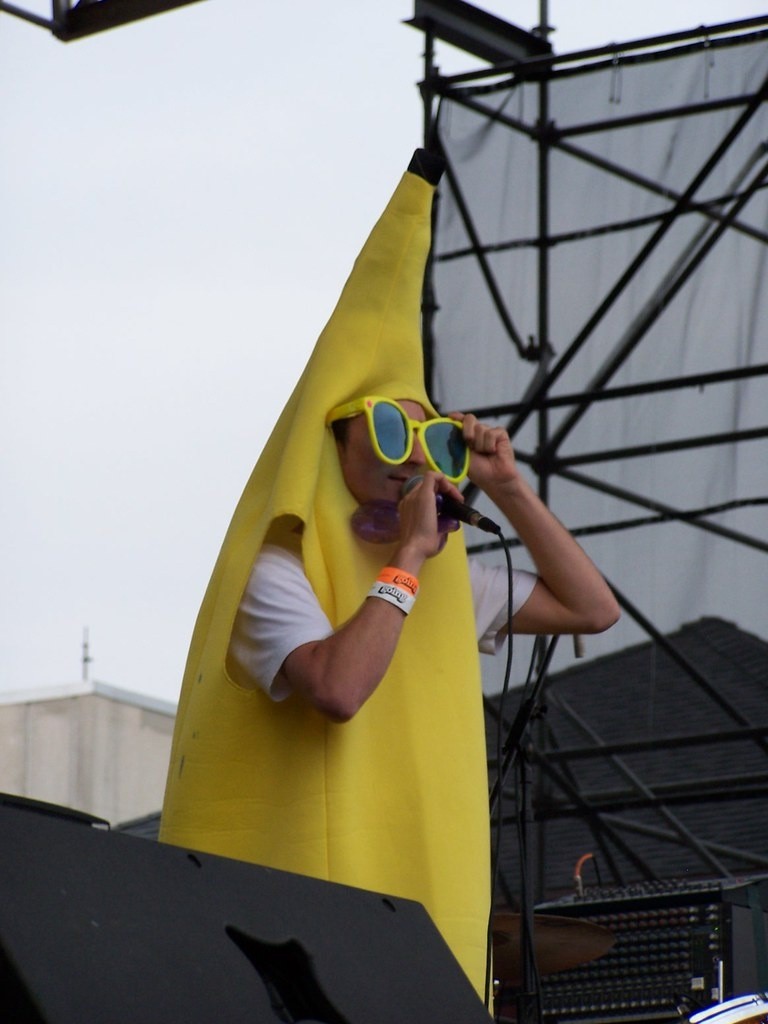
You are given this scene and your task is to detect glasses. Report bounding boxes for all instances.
[327,397,470,482]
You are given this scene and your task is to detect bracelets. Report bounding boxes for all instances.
[368,581,416,615]
[377,567,419,597]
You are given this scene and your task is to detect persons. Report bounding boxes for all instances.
[230,380,622,723]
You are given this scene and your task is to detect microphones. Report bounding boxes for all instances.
[402,474,501,535]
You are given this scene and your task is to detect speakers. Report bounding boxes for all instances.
[0,800,500,1024]
